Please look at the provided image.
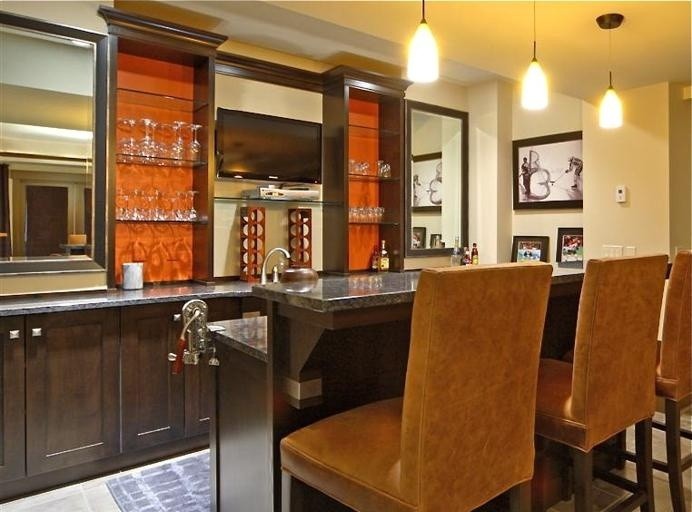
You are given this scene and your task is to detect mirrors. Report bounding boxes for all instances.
[405,100,470,257]
[1,10,110,299]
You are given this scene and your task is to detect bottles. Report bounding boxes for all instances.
[378,240,390,272]
[371,244,381,272]
[450,236,479,267]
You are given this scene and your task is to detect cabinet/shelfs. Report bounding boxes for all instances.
[121,299,209,469]
[205,293,264,323]
[98,5,229,284]
[320,64,413,270]
[1,309,121,503]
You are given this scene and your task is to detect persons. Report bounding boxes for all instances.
[564,155,582,190]
[521,157,531,196]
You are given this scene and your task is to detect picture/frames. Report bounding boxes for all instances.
[409,151,442,213]
[554,225,583,262]
[510,233,550,265]
[510,129,583,213]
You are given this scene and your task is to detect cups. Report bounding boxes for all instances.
[120,263,143,291]
[348,206,387,225]
[349,158,392,178]
[347,274,382,294]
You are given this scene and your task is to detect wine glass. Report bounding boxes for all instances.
[114,183,202,222]
[116,117,202,168]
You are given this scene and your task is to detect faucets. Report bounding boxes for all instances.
[260,246,291,285]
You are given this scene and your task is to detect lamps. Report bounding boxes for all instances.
[597,10,626,131]
[404,3,442,86]
[518,0,552,113]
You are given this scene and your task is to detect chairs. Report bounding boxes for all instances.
[522,248,671,511]
[279,258,555,512]
[564,247,692,511]
